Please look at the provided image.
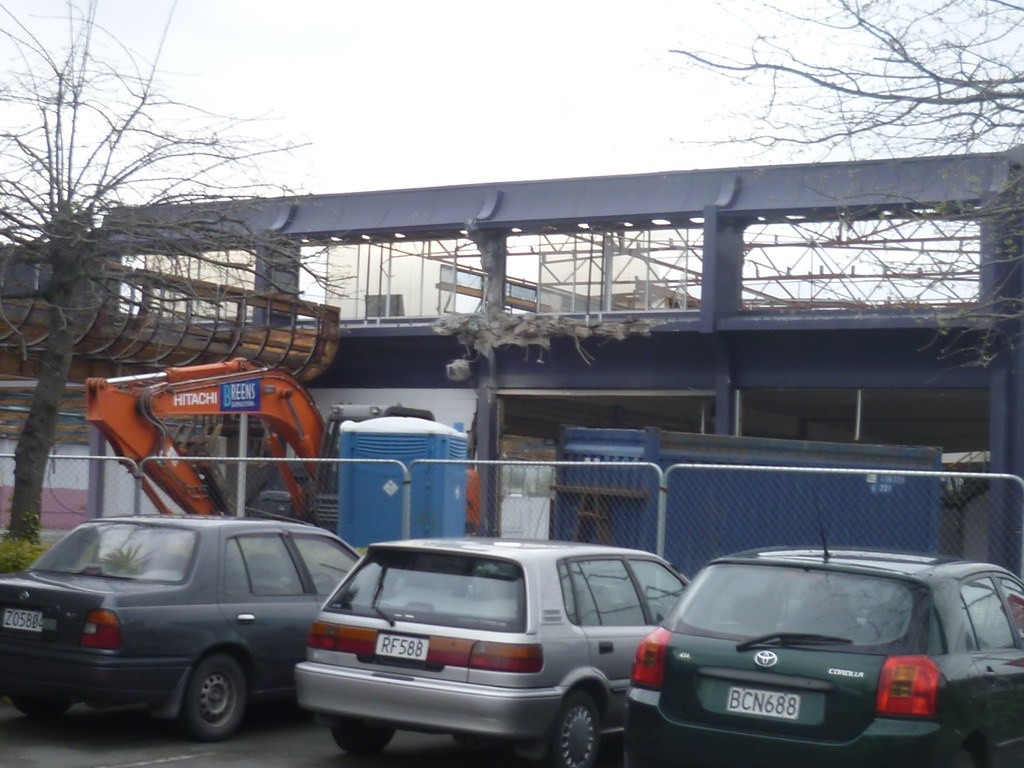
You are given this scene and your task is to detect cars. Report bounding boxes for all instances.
[0,515,363,741]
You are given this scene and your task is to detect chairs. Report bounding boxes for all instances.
[791,578,864,641]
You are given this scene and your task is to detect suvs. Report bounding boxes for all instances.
[295,536,691,768]
[624,493,1024,768]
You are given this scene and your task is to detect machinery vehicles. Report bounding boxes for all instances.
[84,358,481,542]
